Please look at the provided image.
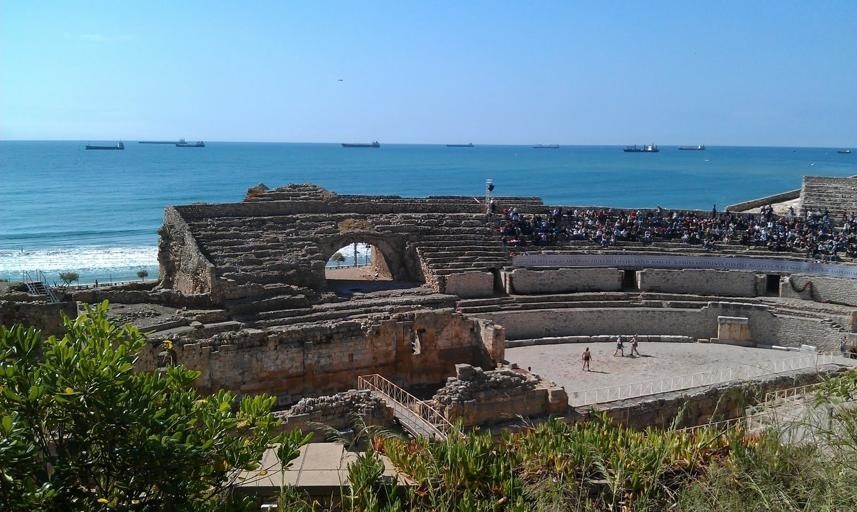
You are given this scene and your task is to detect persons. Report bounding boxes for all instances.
[581,347,592,371]
[630,334,640,356]
[613,334,625,357]
[484,199,857,265]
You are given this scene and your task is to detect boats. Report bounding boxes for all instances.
[446,142,474,147]
[838,149,851,153]
[679,143,705,150]
[532,144,559,148]
[176,141,205,147]
[624,144,659,152]
[86,142,124,149]
[341,140,380,147]
